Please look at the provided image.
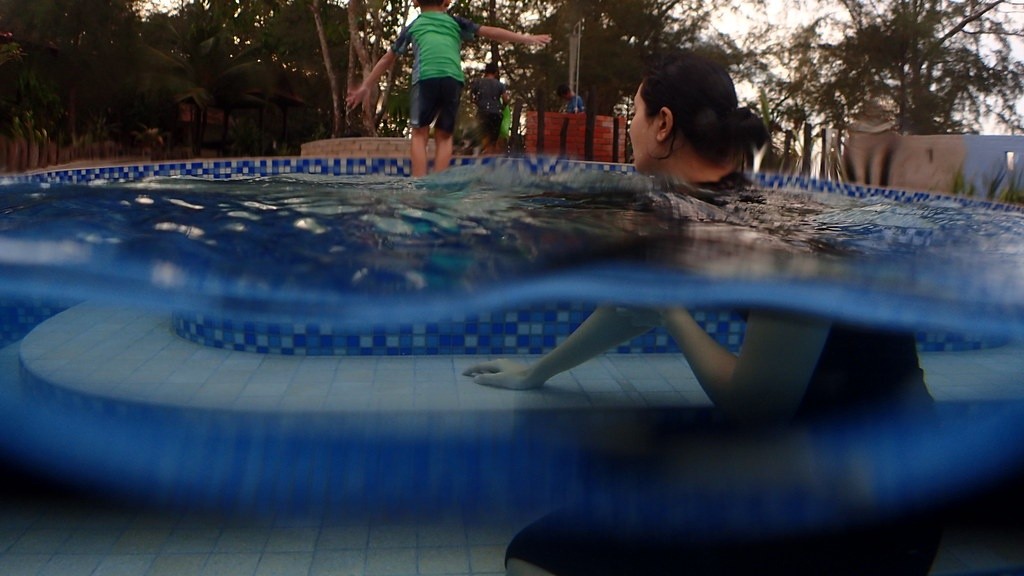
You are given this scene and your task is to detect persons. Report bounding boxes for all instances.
[556,82,584,115]
[462,46,951,576]
[468,63,508,155]
[346,0,554,177]
